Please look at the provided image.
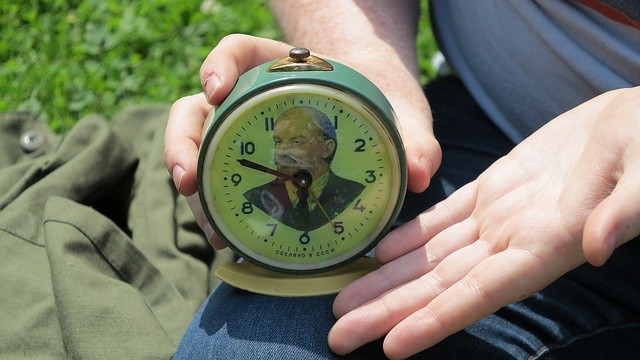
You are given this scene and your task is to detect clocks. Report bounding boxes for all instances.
[195,49,410,299]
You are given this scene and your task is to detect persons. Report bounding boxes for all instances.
[242,106,366,232]
[162,0,640,360]
